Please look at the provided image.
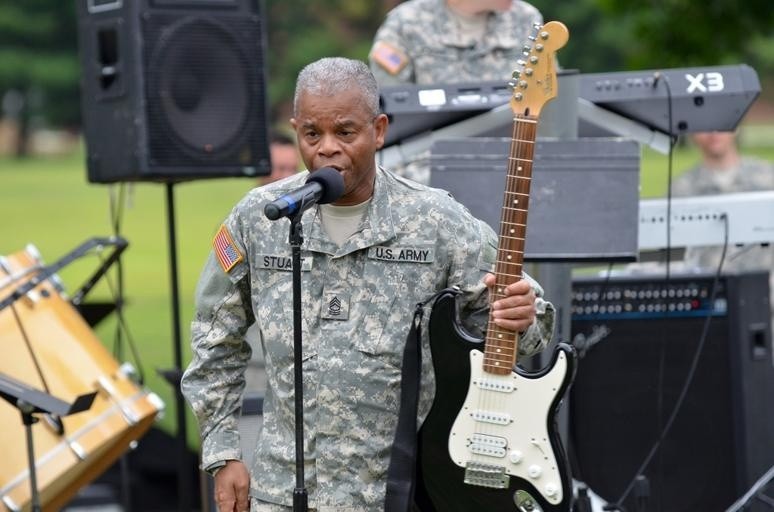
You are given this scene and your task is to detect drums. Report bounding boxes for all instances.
[0,242,166,512]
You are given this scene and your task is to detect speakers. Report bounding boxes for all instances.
[566,273,773,511]
[68,0,278,181]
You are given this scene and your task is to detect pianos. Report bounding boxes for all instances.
[638,191,773,250]
[376,63,763,153]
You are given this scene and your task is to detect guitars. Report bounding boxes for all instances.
[413,20,578,512]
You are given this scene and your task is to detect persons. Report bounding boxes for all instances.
[671,130,773,197]
[366,0,542,85]
[259,130,299,185]
[181,57,557,512]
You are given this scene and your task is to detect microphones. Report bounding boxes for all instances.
[578,319,611,356]
[263,165,347,221]
